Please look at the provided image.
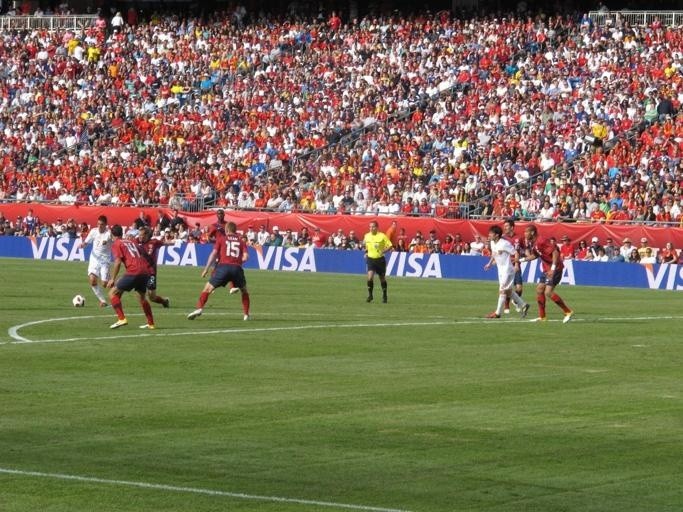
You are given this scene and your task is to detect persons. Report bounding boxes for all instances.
[0,1,681,229]
[0,210,683,332]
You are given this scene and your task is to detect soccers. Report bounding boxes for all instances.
[73,294,86,307]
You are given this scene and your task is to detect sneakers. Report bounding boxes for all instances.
[186,308,202,319]
[230,287,239,294]
[512,299,520,313]
[110,319,127,329]
[244,315,255,321]
[164,297,169,307]
[563,312,574,323]
[532,318,546,321]
[521,305,529,319]
[485,313,499,317]
[140,324,155,330]
[367,295,373,302]
